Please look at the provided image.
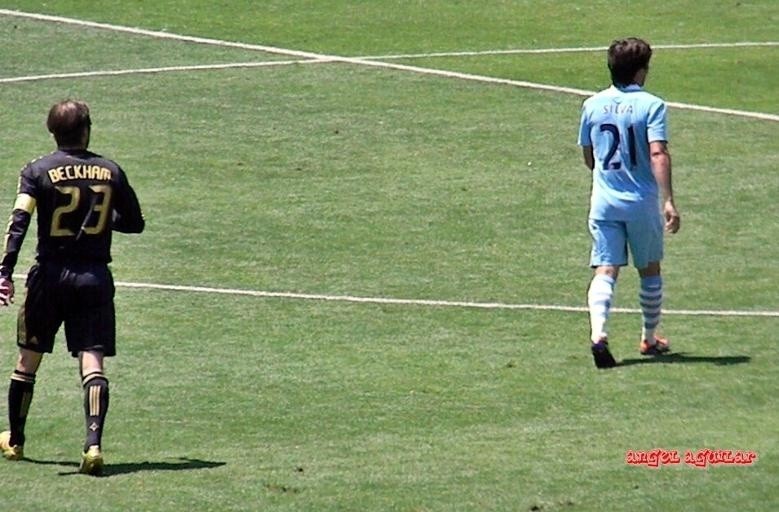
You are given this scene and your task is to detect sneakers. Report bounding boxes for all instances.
[639,336,668,355]
[590,335,616,368]
[79,442,102,474]
[0,431,25,460]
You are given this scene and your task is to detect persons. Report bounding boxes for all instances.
[577,37,680,368]
[0,99,145,473]
